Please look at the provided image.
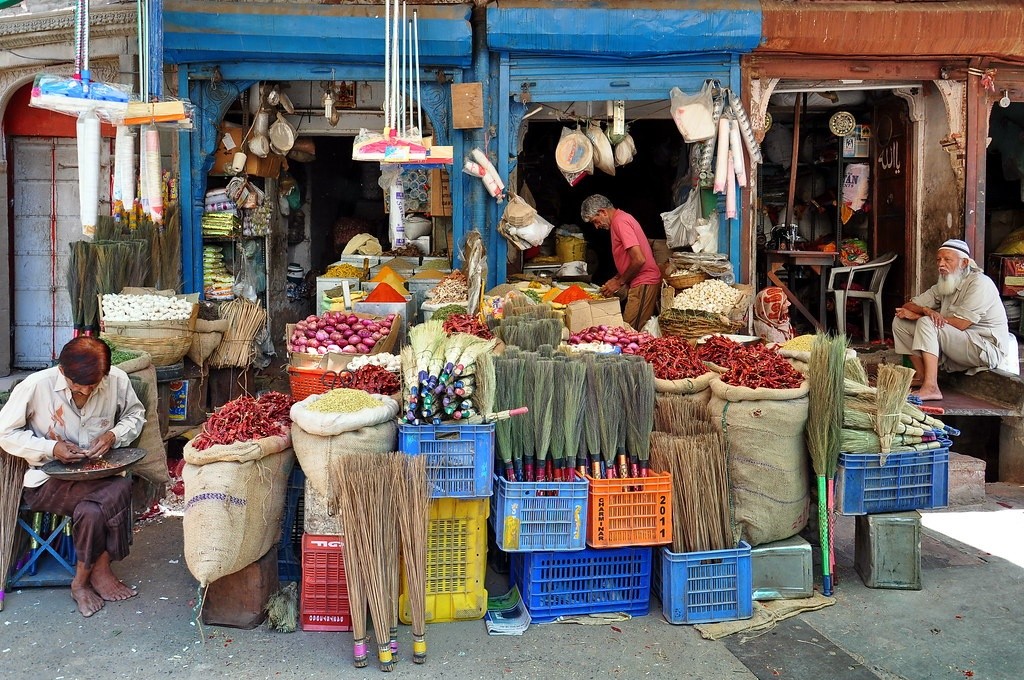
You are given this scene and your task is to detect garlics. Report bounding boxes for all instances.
[347,352,401,372]
[102,292,192,321]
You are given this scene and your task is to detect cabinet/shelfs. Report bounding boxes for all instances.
[811,125,878,267]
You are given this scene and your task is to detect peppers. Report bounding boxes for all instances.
[636,335,805,389]
[193,363,402,453]
[442,314,493,340]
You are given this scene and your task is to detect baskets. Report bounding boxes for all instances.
[666,271,708,289]
[100,331,194,367]
[286,367,351,403]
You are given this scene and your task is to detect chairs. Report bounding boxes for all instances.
[825,250,898,349]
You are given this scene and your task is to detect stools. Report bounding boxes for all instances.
[2,501,77,594]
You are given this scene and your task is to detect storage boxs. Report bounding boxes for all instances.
[273,421,752,631]
[209,126,284,179]
[286,248,459,402]
[834,447,949,514]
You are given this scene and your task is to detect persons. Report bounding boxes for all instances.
[892,239,1020,401]
[0,336,148,617]
[581,194,662,332]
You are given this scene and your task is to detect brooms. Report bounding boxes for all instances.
[65,204,188,339]
[491,343,656,497]
[0,422,77,612]
[648,390,735,565]
[485,296,562,353]
[839,356,961,463]
[803,323,854,596]
[399,318,529,441]
[260,580,299,633]
[325,449,446,672]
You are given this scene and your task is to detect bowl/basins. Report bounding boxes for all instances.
[249,135,269,158]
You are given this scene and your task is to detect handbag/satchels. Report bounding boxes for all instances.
[670,80,716,143]
[232,254,260,305]
[660,184,703,250]
[841,163,870,212]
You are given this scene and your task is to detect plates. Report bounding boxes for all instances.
[269,123,295,150]
[556,126,634,174]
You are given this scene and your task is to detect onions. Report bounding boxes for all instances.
[288,312,395,354]
[570,325,649,354]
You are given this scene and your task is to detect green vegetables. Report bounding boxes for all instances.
[100,338,137,365]
[521,289,541,304]
[430,305,467,320]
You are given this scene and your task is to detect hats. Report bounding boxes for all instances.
[938,239,970,258]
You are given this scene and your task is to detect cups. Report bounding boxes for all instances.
[77,118,163,226]
[233,152,247,172]
